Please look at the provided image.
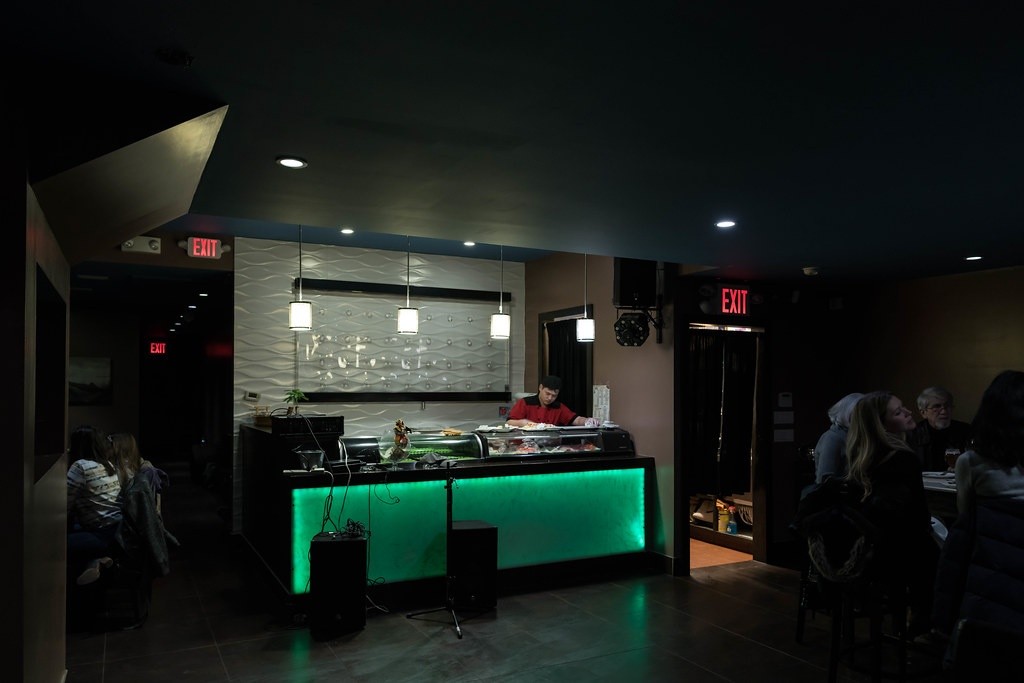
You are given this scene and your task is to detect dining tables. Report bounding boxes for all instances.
[921,476,957,493]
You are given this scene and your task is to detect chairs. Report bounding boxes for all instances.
[88,474,153,631]
[791,440,1024,683]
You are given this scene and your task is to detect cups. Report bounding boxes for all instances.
[945,449,961,467]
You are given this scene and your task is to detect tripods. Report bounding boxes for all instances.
[406,461,486,639]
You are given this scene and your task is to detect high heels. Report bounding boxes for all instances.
[77,556,113,585]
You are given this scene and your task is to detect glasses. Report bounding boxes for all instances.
[926,406,954,413]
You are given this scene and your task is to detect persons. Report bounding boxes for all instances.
[505,376,587,426]
[837,392,935,644]
[814,393,865,484]
[67,424,170,629]
[907,386,972,469]
[935,371,1024,669]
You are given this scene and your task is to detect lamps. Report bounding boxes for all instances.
[121,235,161,253]
[288,225,313,332]
[398,236,419,336]
[490,244,511,340]
[576,253,595,342]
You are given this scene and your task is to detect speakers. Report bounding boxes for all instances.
[613,256,657,307]
[452,520,498,600]
[309,532,368,643]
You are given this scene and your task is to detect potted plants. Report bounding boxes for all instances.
[282,388,310,416]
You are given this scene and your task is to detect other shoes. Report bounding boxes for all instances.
[906,608,929,627]
